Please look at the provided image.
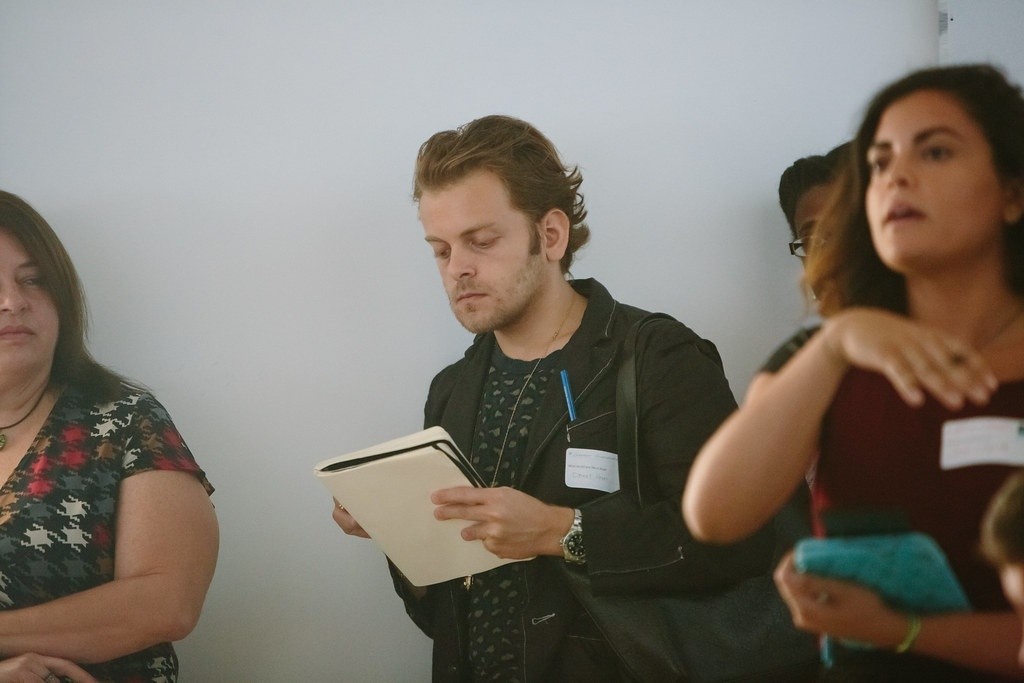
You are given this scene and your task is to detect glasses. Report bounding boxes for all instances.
[788,230,828,258]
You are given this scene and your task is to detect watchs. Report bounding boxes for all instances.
[560,507,586,566]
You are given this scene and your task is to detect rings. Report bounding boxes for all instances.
[43,674,59,683]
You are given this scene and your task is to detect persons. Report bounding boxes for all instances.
[678,63,1024,683]
[314,115,823,683]
[0,190,219,682]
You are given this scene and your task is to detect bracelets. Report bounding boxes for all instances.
[896,610,921,652]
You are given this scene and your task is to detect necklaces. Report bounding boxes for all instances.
[1,374,53,451]
[462,291,576,592]
[971,304,1022,349]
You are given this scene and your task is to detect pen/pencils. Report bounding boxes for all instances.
[560,369,577,422]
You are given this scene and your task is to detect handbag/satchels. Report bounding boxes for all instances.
[560,312,820,683]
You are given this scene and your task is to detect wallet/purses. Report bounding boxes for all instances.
[793,533,973,670]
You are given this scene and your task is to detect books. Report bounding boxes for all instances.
[312,426,537,587]
